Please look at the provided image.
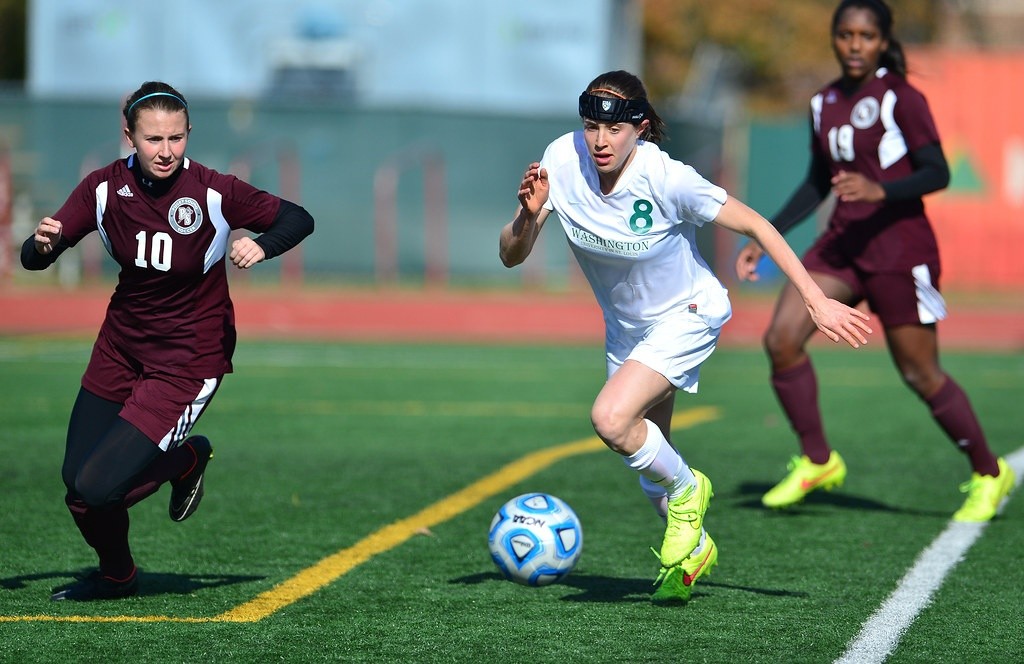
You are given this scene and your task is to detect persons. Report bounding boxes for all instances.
[736,0,1011,524]
[498,72,873,603]
[19,80,314,603]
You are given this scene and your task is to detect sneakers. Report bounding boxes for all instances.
[659,468,715,569]
[650,532,720,607]
[167,433,215,523]
[951,457,1016,526]
[53,569,144,607]
[760,450,848,511]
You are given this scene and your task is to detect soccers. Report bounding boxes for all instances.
[487,493,584,585]
